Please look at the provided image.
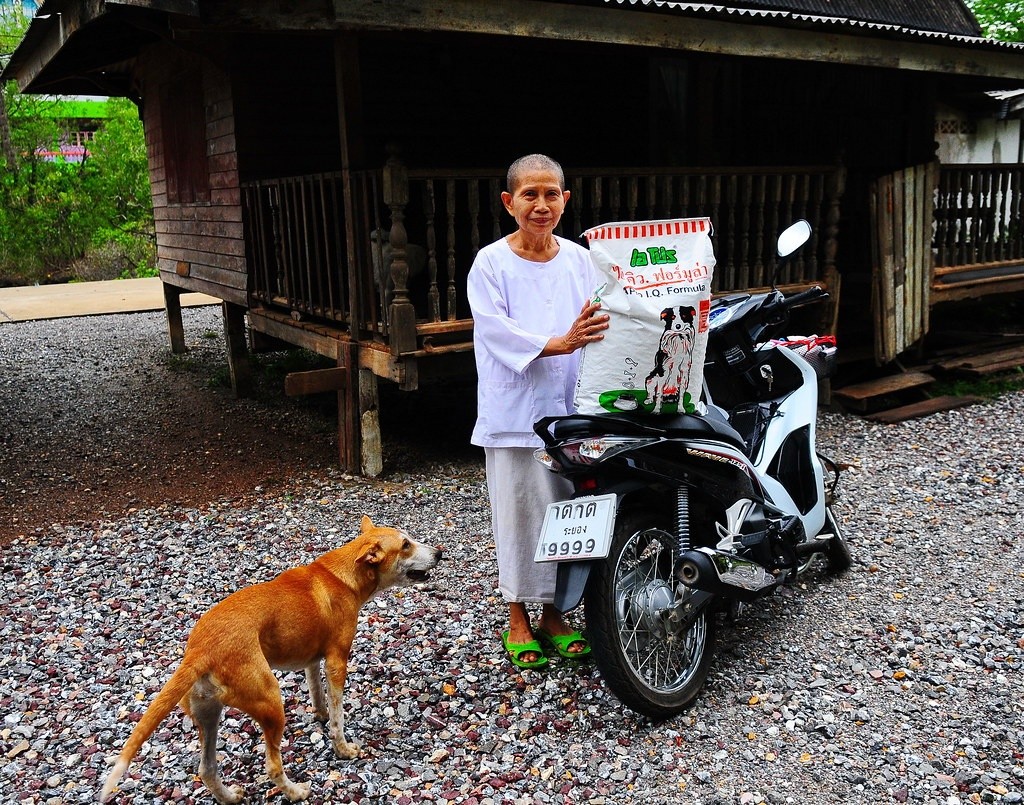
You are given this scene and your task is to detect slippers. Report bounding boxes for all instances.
[538,619,592,659]
[501,626,549,670]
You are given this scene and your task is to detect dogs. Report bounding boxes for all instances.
[93,515,443,803]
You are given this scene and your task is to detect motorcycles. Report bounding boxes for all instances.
[529,215,856,722]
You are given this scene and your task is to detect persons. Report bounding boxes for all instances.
[466,154,612,668]
